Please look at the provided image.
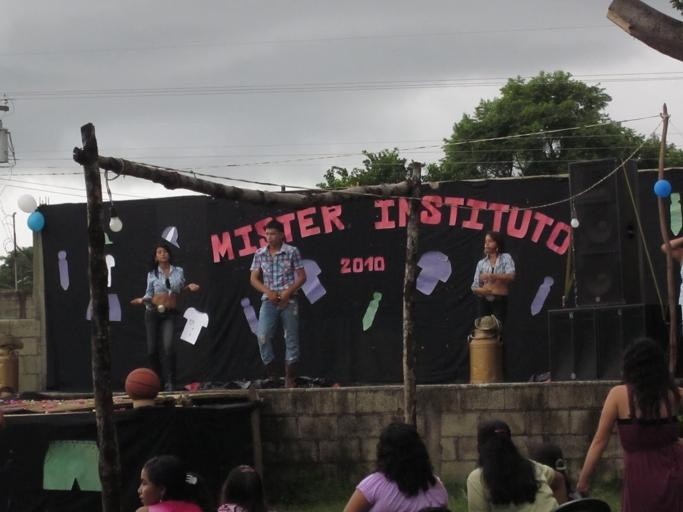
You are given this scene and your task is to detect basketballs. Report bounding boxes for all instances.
[126,368,160,400]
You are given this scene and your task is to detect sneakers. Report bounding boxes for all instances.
[262,374,299,388]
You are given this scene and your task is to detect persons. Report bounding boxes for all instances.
[130,245,199,390]
[134,456,263,512]
[577,336,683,512]
[250,220,306,388]
[467,420,570,512]
[661,237,683,324]
[471,233,516,342]
[343,423,448,512]
